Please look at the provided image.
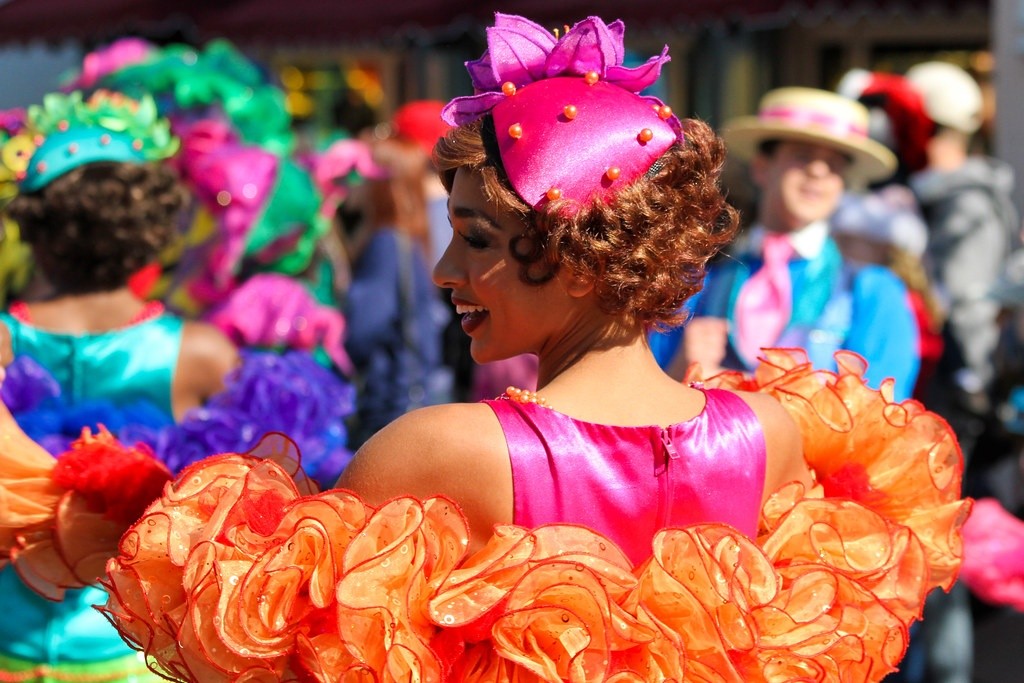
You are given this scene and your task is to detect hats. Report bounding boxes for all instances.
[723,86,897,180]
[902,60,985,134]
[390,98,456,158]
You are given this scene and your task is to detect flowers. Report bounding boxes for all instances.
[440,13,672,126]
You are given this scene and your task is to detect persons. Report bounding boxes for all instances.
[643,86,921,405]
[830,65,948,358]
[311,99,541,455]
[92,13,977,683]
[901,59,1019,683]
[0,90,357,683]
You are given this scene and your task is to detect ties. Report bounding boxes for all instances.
[734,235,797,364]
